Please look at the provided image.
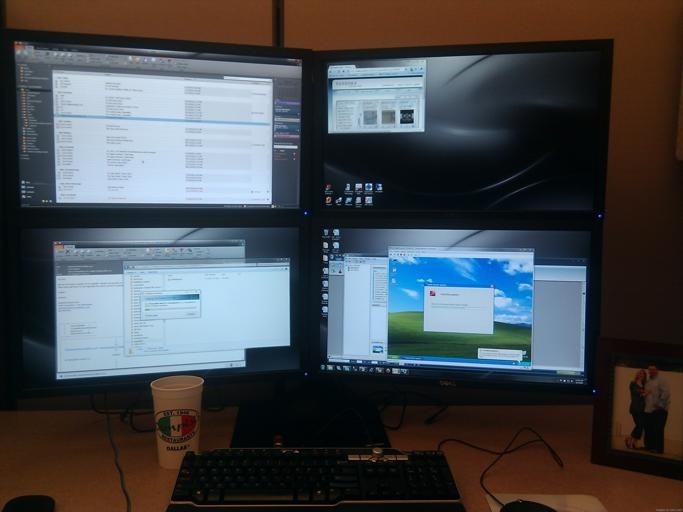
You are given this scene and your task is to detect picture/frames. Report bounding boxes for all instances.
[591,337,683,481]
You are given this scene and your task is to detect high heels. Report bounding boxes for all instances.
[624,437,641,449]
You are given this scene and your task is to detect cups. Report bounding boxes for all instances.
[149,375,205,471]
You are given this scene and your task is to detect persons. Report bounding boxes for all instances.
[623,369,649,448]
[642,365,670,454]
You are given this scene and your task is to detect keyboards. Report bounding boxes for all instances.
[165,445,465,512]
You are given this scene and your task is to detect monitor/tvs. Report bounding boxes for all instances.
[313,39,616,216]
[0,28,312,222]
[306,216,605,410]
[0,218,306,412]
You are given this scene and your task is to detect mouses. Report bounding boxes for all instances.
[498,498,555,512]
